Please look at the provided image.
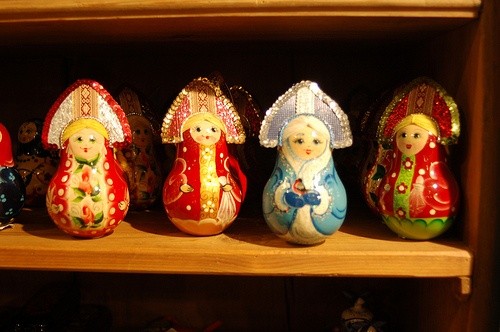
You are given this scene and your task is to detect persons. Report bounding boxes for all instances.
[161,77,247,235]
[119,86,160,210]
[11,119,60,208]
[365,78,460,240]
[258,81,353,245]
[42,80,132,239]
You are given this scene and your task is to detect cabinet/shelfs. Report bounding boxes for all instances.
[0,0,500,332]
